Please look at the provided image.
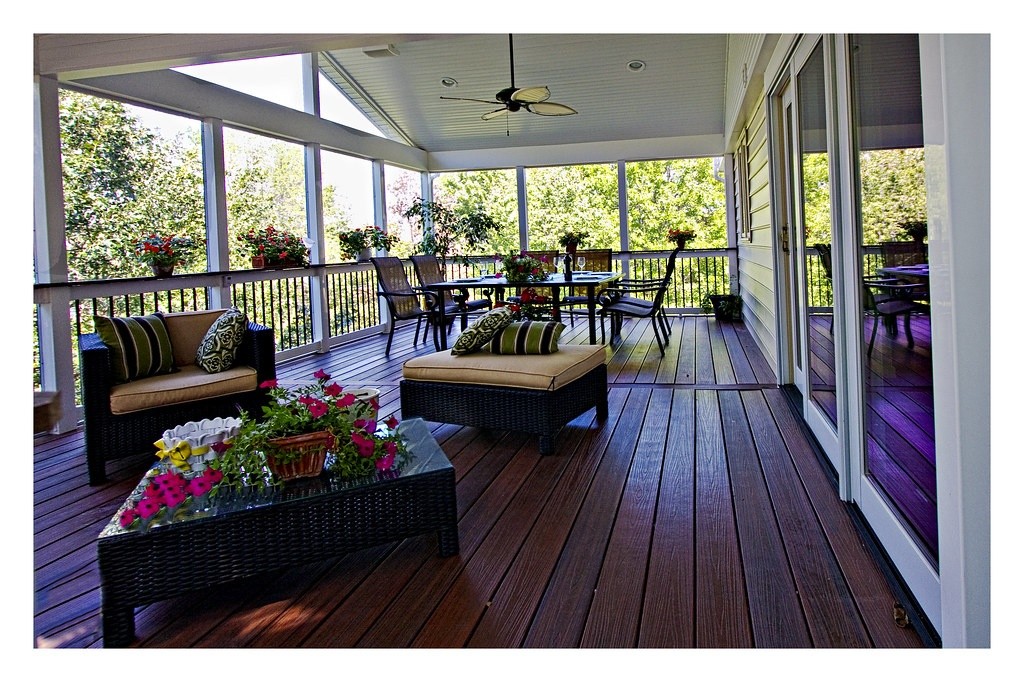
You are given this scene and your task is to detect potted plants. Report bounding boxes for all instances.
[700,285,744,323]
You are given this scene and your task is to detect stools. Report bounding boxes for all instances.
[400,344,608,455]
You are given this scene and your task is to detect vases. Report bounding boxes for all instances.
[265,253,301,270]
[911,231,924,242]
[676,238,685,249]
[149,259,175,277]
[261,428,328,481]
[558,231,591,252]
[355,246,372,262]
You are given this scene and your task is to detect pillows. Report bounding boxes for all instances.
[483,318,568,356]
[193,305,247,373]
[450,303,519,356]
[92,311,181,386]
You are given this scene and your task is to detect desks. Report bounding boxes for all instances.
[874,264,929,350]
[97,416,458,649]
[427,269,620,345]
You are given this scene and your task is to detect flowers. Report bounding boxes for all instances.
[337,225,401,260]
[237,226,312,267]
[131,232,207,266]
[204,370,410,497]
[666,228,697,244]
[897,220,927,236]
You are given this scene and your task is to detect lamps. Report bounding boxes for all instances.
[438,34,577,137]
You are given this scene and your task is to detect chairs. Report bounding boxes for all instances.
[814,243,927,354]
[368,257,458,358]
[77,311,275,485]
[516,247,681,358]
[409,255,494,336]
